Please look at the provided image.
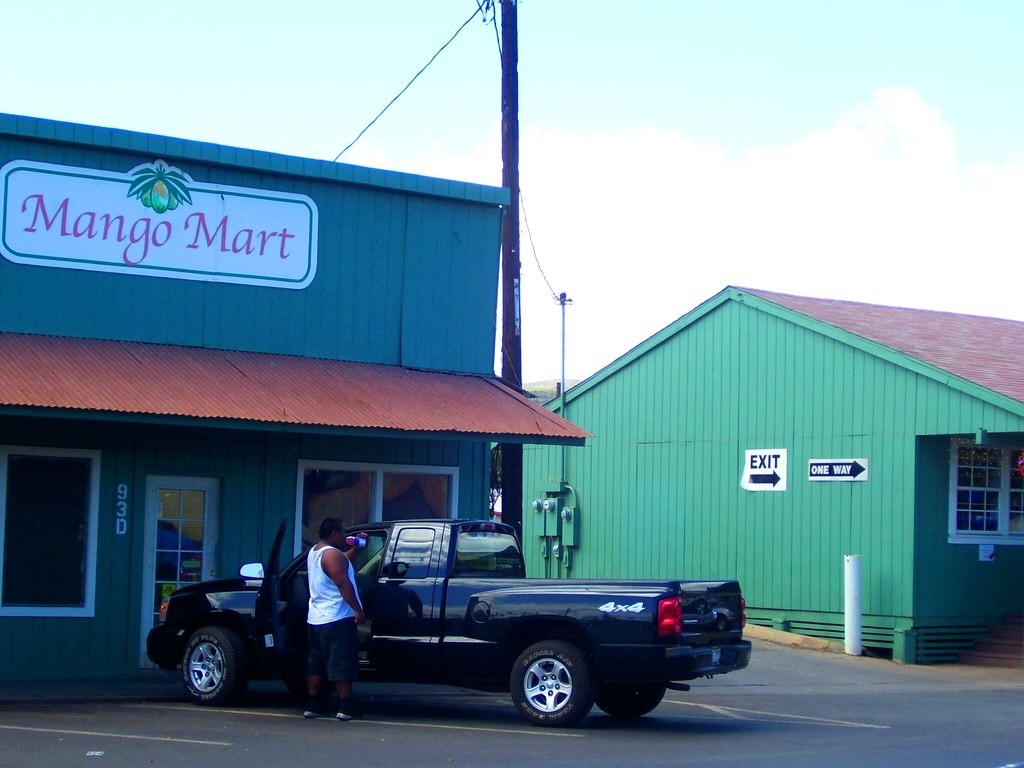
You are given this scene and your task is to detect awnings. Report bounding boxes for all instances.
[0,329,594,447]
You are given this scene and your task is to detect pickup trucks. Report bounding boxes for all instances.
[146,519,753,729]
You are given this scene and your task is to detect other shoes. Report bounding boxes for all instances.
[303,704,336,717]
[336,708,366,721]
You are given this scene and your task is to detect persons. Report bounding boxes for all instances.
[302,516,368,721]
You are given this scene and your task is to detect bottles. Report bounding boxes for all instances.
[345,536,367,548]
